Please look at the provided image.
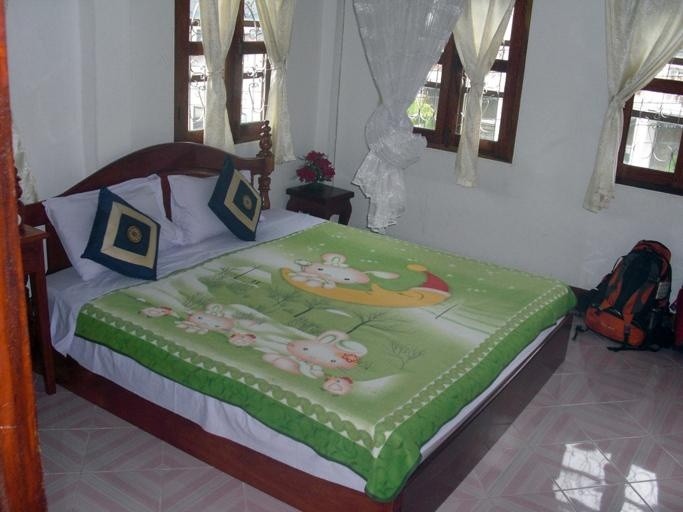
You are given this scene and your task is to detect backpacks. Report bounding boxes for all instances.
[572,240,672,352]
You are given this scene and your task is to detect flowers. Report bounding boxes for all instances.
[291,149,336,183]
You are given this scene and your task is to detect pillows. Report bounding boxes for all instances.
[38,153,265,283]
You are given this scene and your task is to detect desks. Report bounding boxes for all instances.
[17,221,56,395]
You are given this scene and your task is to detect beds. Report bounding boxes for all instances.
[11,119,577,512]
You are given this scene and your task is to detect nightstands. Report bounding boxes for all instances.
[286,183,355,227]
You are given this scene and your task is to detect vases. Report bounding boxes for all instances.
[307,180,323,192]
